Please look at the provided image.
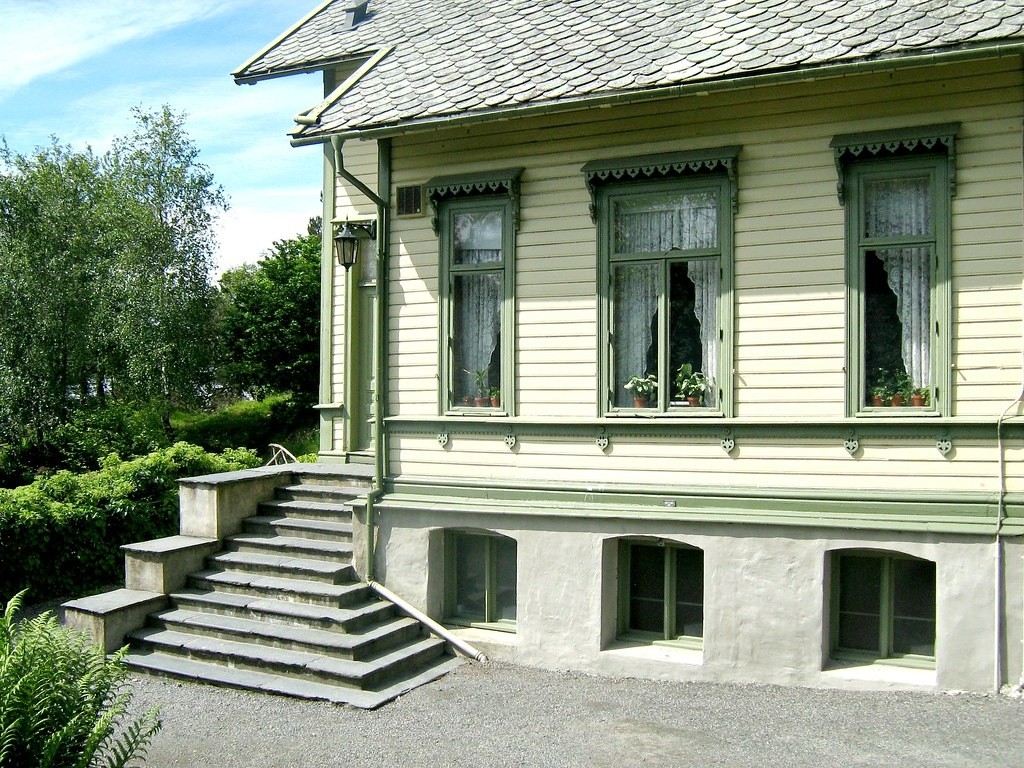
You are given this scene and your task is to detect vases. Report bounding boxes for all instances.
[463,395,473,406]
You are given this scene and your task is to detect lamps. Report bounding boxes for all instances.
[333,215,376,272]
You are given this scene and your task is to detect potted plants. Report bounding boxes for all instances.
[909,388,927,405]
[885,369,911,406]
[463,366,491,407]
[624,373,658,407]
[675,363,710,407]
[486,386,499,407]
[868,386,887,406]
